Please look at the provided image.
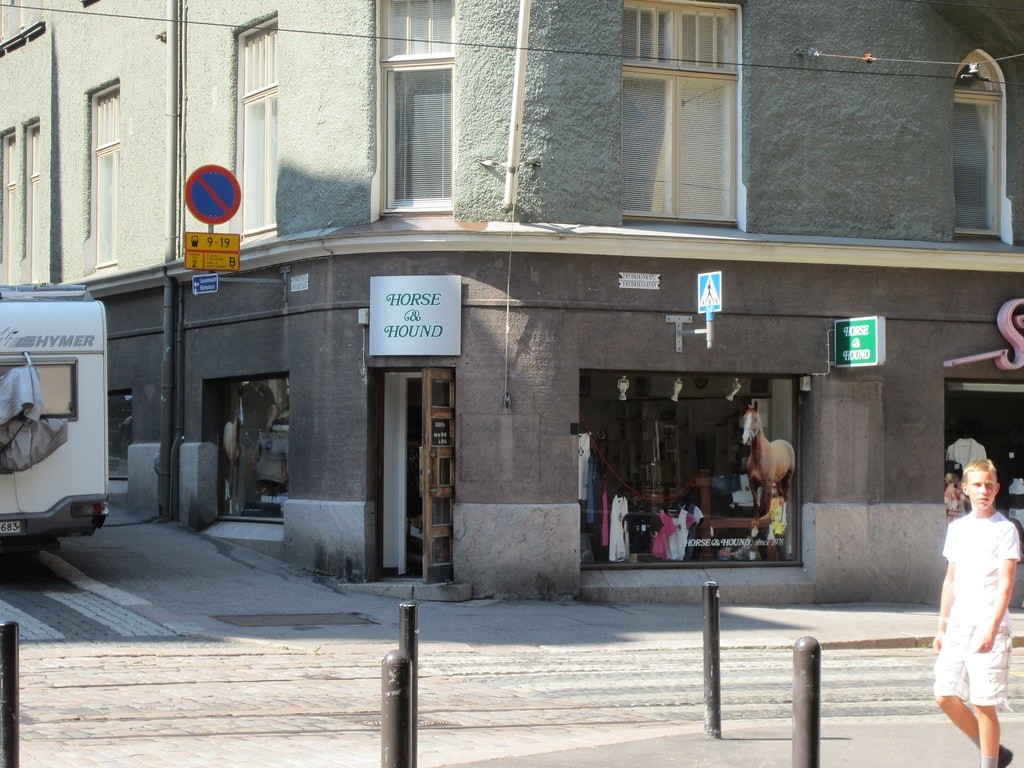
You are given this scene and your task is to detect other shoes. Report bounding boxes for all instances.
[996,746,1013,768]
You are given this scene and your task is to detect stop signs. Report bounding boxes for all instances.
[185,164,242,224]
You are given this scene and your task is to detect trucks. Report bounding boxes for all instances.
[0,282,114,569]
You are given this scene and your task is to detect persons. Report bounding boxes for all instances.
[236,380,279,512]
[932,460,1022,768]
[754,479,790,558]
[944,472,973,527]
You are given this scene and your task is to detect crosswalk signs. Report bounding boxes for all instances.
[697,271,723,315]
